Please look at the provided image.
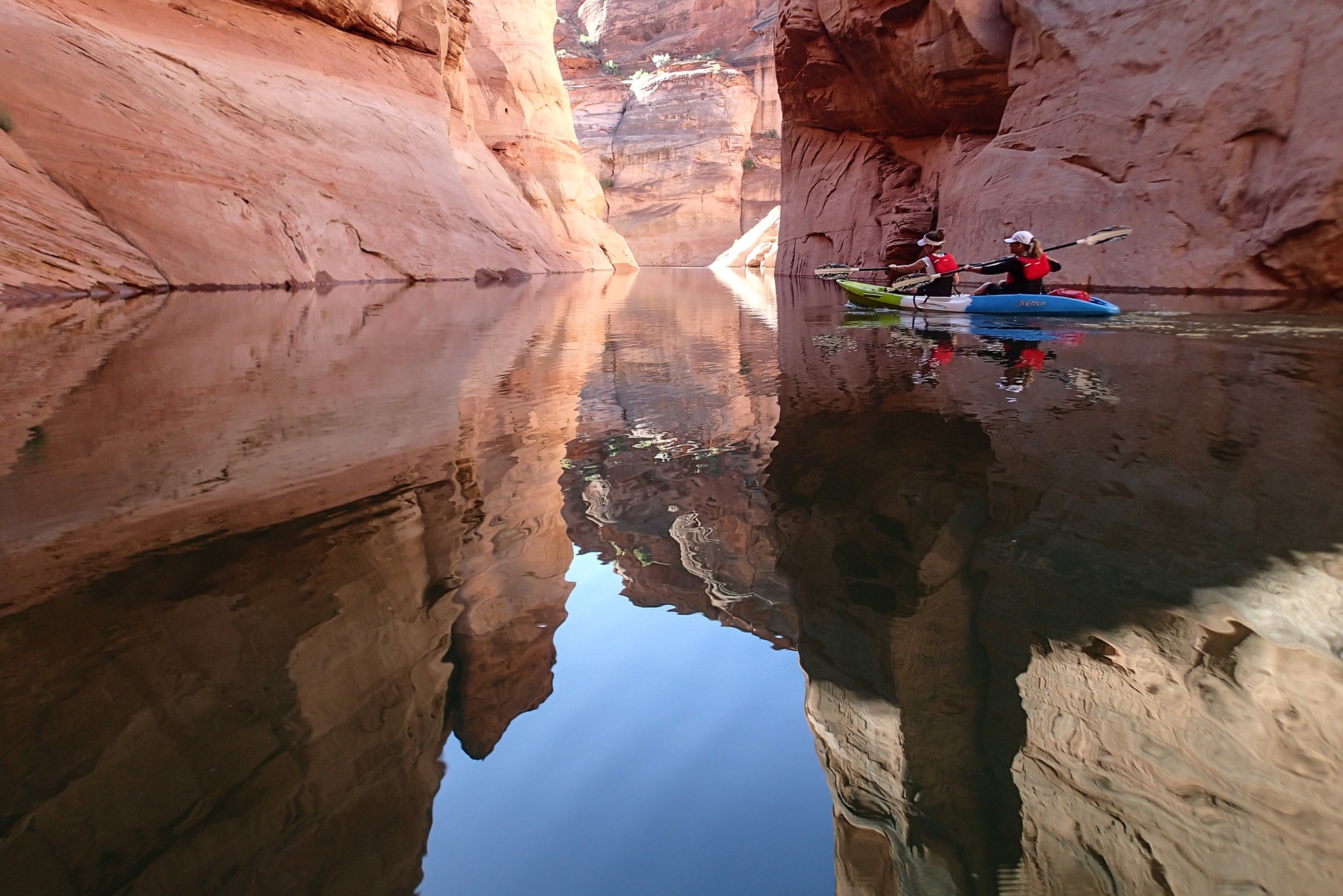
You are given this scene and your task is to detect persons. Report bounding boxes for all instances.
[963,231,1062,296]
[888,227,960,297]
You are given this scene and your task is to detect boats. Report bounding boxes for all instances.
[835,278,1121,318]
[837,306,1121,334]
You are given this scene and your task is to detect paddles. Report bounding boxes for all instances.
[893,225,1135,293]
[815,262,991,281]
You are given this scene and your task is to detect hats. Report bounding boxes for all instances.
[1004,231,1035,245]
[917,234,945,246]
